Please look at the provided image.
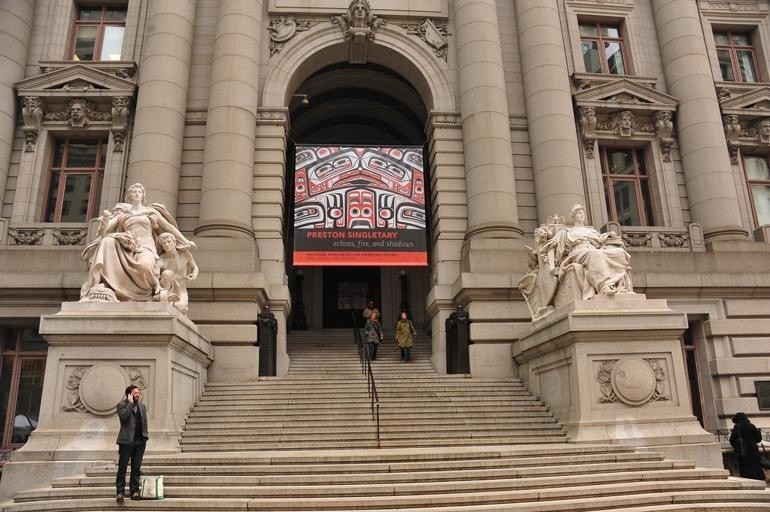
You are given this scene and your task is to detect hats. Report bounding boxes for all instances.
[733,413,750,423]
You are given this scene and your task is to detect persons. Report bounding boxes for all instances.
[348,0,372,28]
[363,299,383,324]
[152,233,200,315]
[365,311,384,363]
[70,99,91,120]
[86,180,179,301]
[616,110,634,128]
[756,115,770,136]
[395,311,417,363]
[115,384,149,501]
[532,203,632,296]
[729,411,767,480]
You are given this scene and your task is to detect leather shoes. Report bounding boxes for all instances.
[131,491,139,499]
[116,495,124,503]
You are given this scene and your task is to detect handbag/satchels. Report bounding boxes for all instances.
[736,438,748,458]
[140,475,164,499]
[379,336,384,343]
[760,456,770,469]
